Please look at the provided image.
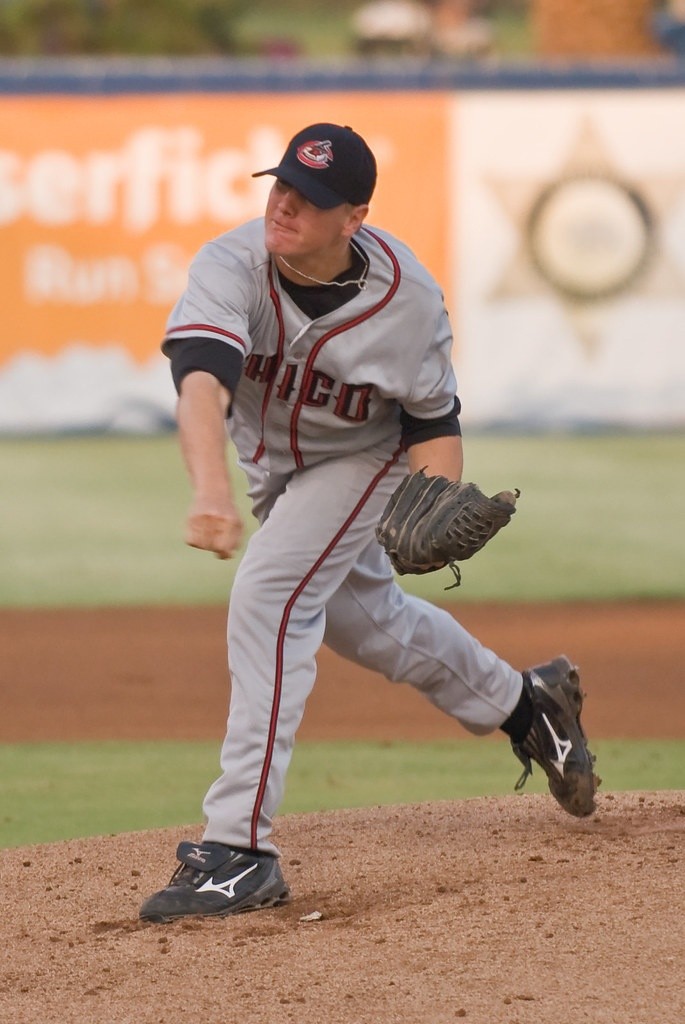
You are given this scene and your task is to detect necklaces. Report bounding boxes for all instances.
[280,241,369,290]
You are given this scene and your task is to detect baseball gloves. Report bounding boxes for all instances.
[375,465,522,591]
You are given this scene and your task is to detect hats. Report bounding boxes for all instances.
[252,122,377,210]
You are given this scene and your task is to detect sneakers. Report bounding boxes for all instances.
[138,837,292,923]
[514,655,603,817]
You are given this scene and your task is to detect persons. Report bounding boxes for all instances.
[137,124,602,923]
